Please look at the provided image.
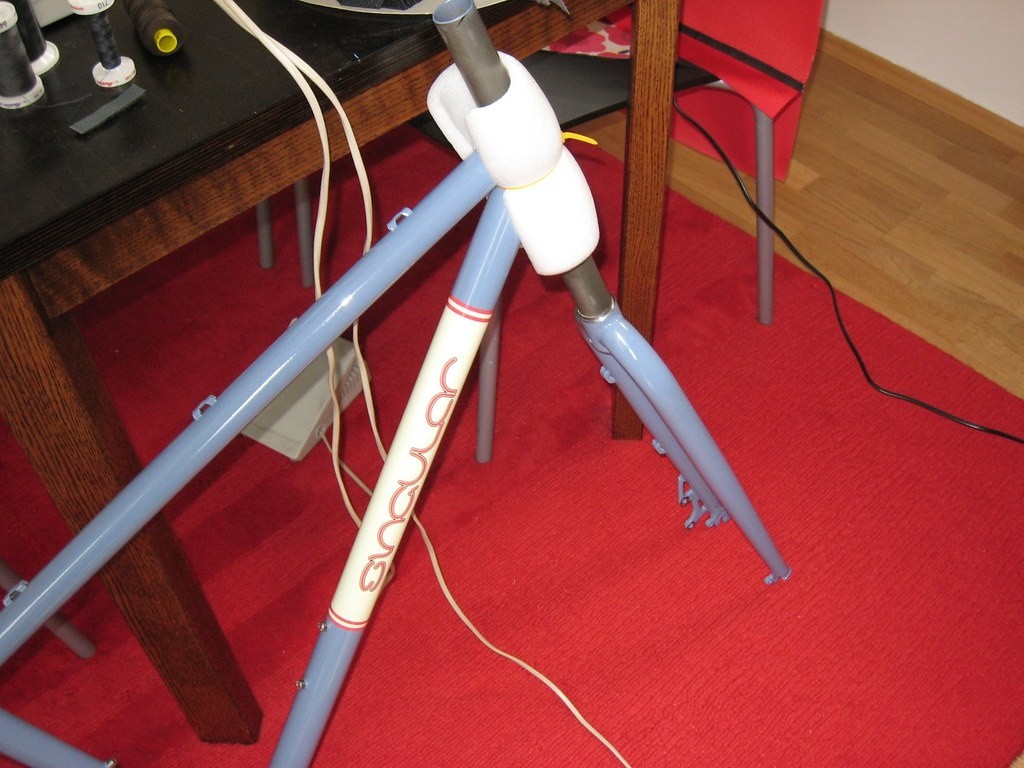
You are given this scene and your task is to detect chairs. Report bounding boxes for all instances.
[295,0,825,465]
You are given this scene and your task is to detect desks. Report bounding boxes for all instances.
[0,0,683,744]
